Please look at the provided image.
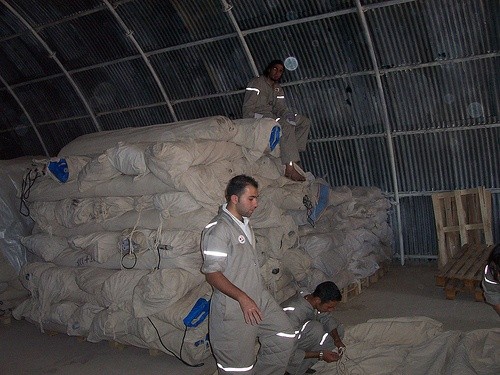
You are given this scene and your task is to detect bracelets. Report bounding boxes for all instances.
[319,350,324,361]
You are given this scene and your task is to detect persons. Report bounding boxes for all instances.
[243,59,311,181]
[258,280,347,375]
[200,175,294,375]
[482,242,500,315]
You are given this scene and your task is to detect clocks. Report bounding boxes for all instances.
[284,57,299,71]
